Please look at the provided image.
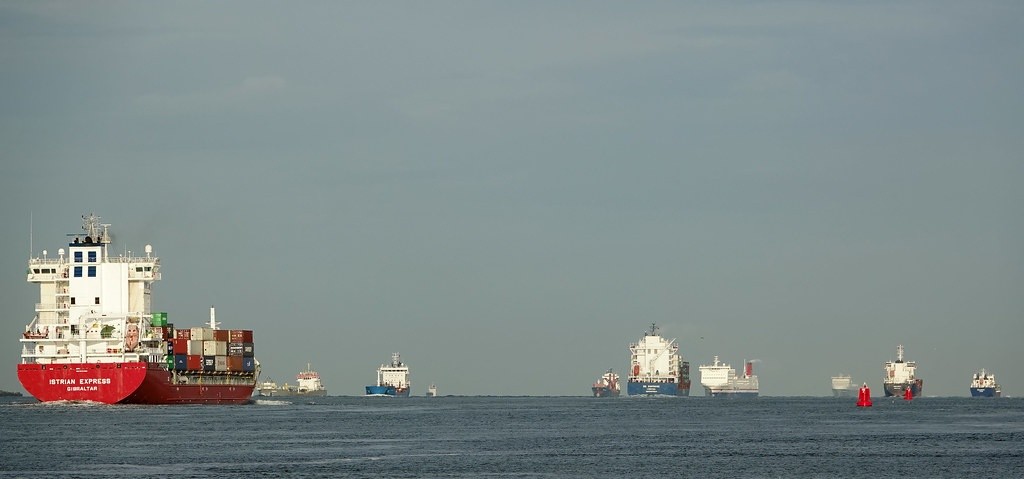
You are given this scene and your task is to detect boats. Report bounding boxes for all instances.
[16,212,261,405]
[883,344,923,397]
[256,361,328,397]
[627,320,691,397]
[365,352,413,397]
[592,368,620,397]
[831,375,860,398]
[969,367,1002,398]
[699,355,759,397]
